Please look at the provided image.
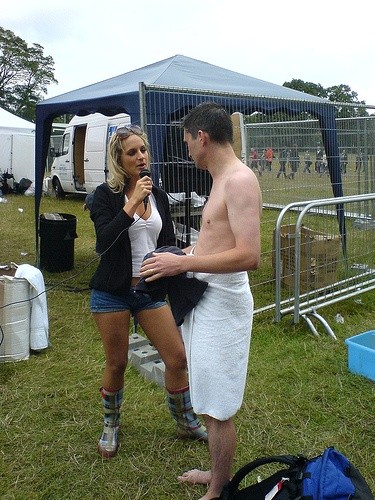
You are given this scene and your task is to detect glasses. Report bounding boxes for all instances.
[113,125,143,141]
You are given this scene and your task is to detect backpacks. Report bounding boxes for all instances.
[210,446,374,500]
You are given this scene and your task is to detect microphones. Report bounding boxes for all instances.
[140,169,151,208]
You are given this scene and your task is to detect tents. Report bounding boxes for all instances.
[0,53,347,271]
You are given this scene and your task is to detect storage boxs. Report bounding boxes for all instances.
[277,222,341,295]
[343,329,375,382]
[0,261,32,364]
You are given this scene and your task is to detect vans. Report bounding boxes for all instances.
[49,115,131,201]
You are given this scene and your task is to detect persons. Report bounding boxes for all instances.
[248,144,370,180]
[138,102,264,500]
[90,125,211,459]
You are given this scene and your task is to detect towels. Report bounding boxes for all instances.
[15,263,49,352]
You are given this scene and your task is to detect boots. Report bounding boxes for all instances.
[164,386,208,442]
[97,385,124,458]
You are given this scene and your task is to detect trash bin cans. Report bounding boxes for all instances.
[39,213,77,271]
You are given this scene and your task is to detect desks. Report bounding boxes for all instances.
[170,210,203,218]
